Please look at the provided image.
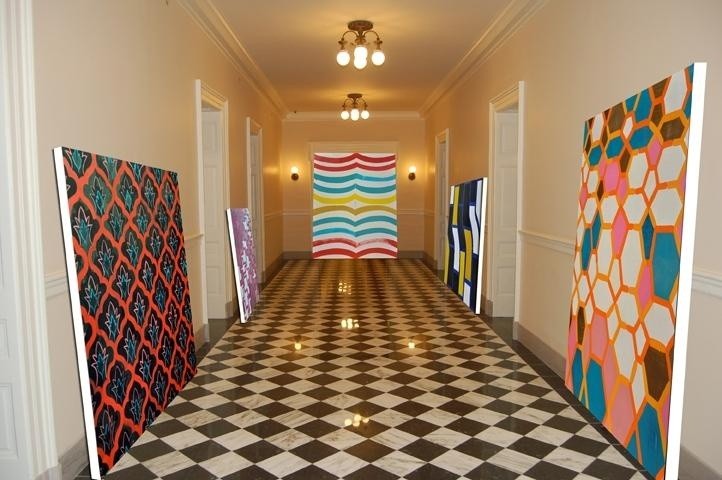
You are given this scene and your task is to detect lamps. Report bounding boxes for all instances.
[340,93,369,122]
[336,21,386,72]
